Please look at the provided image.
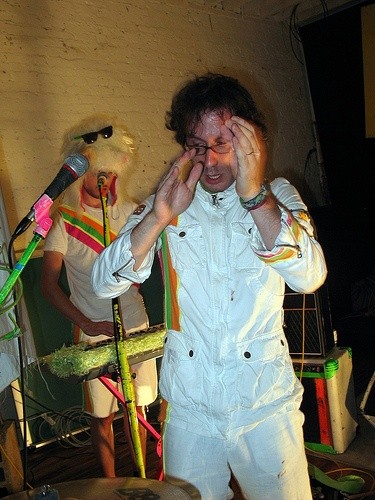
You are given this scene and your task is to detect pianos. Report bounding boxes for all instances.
[49,322,165,381]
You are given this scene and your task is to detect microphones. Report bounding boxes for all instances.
[12,153,89,239]
[97,172,108,186]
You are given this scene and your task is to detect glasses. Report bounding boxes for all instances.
[184,143,232,155]
[74,125,112,144]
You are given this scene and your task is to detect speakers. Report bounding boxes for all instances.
[282,282,336,358]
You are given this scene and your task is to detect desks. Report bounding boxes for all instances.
[0,478,190,500]
